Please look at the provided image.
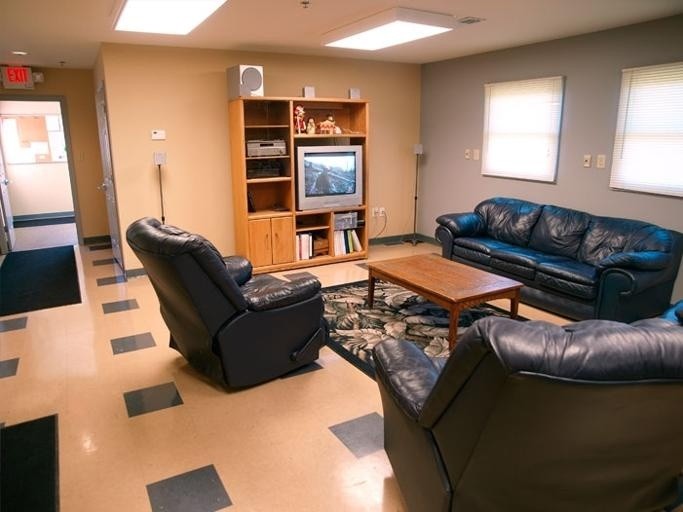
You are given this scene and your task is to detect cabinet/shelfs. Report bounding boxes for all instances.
[231,96,370,274]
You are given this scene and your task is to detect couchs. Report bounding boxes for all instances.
[434,198,683,324]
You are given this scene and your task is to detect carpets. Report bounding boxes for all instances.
[1,245,82,316]
[317,275,532,379]
[3,414,61,512]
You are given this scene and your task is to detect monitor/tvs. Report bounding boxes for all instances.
[296,144,363,212]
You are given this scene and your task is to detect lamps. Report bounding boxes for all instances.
[154,151,168,223]
[404,143,425,246]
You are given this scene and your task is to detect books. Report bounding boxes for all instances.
[248,191,256,212]
[334,230,362,256]
[296,234,312,260]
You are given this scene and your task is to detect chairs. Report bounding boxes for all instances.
[125,216,326,391]
[371,299,683,509]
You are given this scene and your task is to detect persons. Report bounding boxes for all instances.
[294,105,336,134]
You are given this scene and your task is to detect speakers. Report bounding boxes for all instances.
[226,63,264,98]
[153,151,166,166]
[414,143,424,154]
